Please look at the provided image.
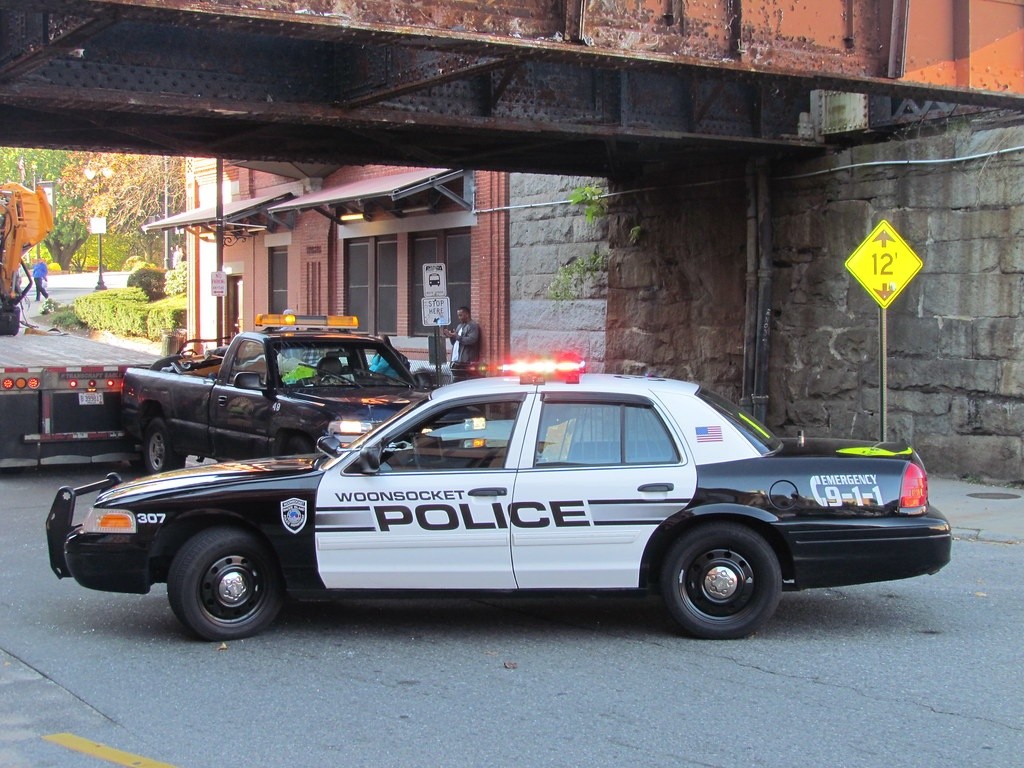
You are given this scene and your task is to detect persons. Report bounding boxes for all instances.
[442,306,481,381]
[32,256,53,304]
[171,244,184,268]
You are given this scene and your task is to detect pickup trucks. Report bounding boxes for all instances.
[121,314,433,474]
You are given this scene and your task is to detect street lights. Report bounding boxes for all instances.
[84,167,113,290]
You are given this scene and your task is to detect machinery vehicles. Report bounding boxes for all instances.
[0,182,54,336]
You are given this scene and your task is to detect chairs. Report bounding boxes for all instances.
[310,356,356,387]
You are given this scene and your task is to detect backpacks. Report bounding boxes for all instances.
[373,347,410,374]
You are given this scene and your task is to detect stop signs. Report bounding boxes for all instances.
[421,297,451,326]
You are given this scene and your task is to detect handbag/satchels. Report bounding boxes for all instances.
[44,280,48,288]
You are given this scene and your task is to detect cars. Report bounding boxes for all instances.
[64,351,953,640]
